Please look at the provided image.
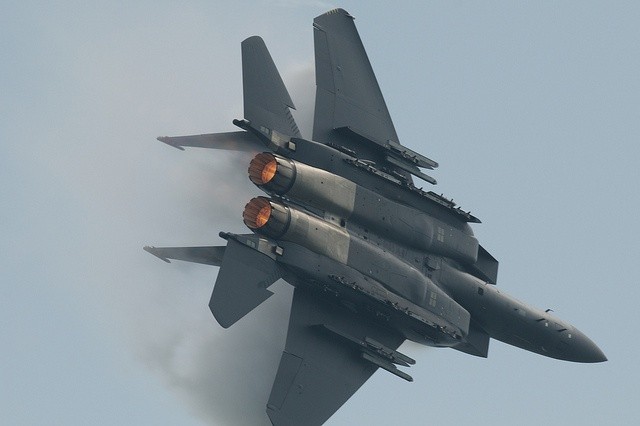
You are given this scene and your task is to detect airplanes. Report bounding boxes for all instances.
[141,7,608,426]
